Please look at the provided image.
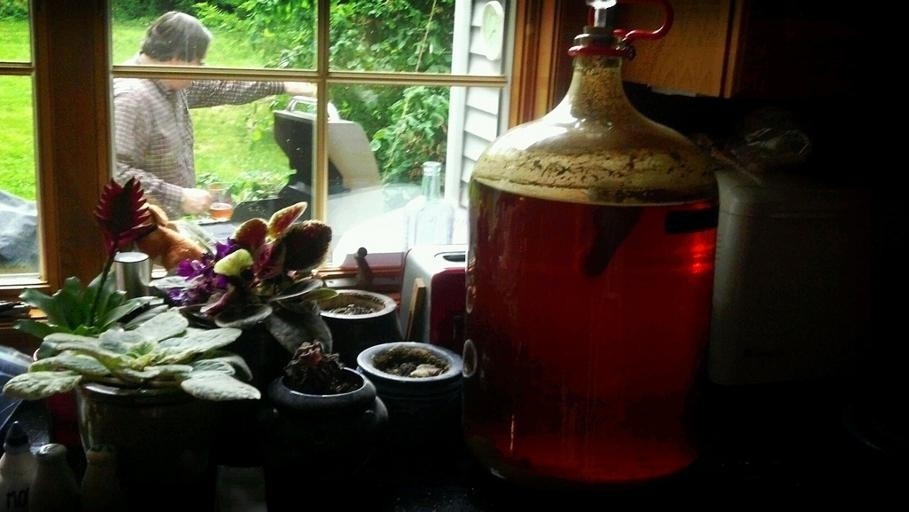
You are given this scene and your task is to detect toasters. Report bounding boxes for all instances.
[397,247,467,353]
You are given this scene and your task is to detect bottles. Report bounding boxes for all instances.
[405,163,456,246]
[0,421,123,512]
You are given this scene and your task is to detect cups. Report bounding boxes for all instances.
[208,182,233,223]
[113,252,152,298]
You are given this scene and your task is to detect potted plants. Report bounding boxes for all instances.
[19,311,462,494]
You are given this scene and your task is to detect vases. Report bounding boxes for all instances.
[315,288,401,354]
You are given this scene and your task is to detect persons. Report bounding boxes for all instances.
[112,9,317,221]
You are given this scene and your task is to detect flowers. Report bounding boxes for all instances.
[167,200,335,318]
[77,176,162,326]
